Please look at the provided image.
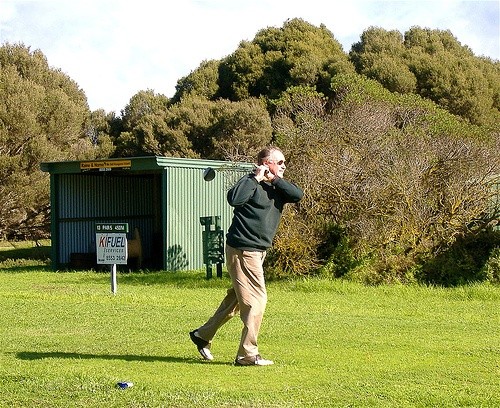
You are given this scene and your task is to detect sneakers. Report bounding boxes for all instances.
[189,329,214,360]
[236,355,274,366]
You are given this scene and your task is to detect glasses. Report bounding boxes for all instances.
[268,159,287,165]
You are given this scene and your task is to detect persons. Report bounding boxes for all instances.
[189,145,303,366]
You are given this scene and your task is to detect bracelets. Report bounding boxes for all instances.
[271,174,278,182]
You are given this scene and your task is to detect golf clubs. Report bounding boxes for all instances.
[203,167,270,181]
